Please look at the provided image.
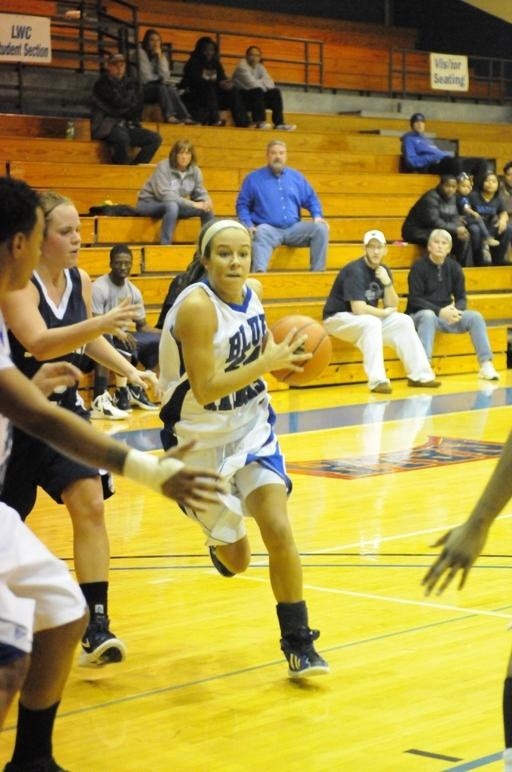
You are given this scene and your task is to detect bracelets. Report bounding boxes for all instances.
[383,279,393,288]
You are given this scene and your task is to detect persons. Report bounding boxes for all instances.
[92,26,298,166]
[154,215,332,679]
[405,227,499,382]
[59,350,130,418]
[91,242,164,413]
[0,190,166,667]
[319,228,442,394]
[396,112,512,267]
[418,429,512,772]
[134,135,330,273]
[0,177,228,772]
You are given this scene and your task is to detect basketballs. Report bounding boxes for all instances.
[263,315,331,383]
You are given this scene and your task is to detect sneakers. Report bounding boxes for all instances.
[78,614,125,668]
[276,124,297,131]
[479,362,499,380]
[281,625,330,677]
[375,383,392,393]
[90,384,157,420]
[258,120,272,129]
[408,379,441,387]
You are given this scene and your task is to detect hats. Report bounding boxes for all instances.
[108,54,126,62]
[410,113,427,128]
[364,230,385,247]
